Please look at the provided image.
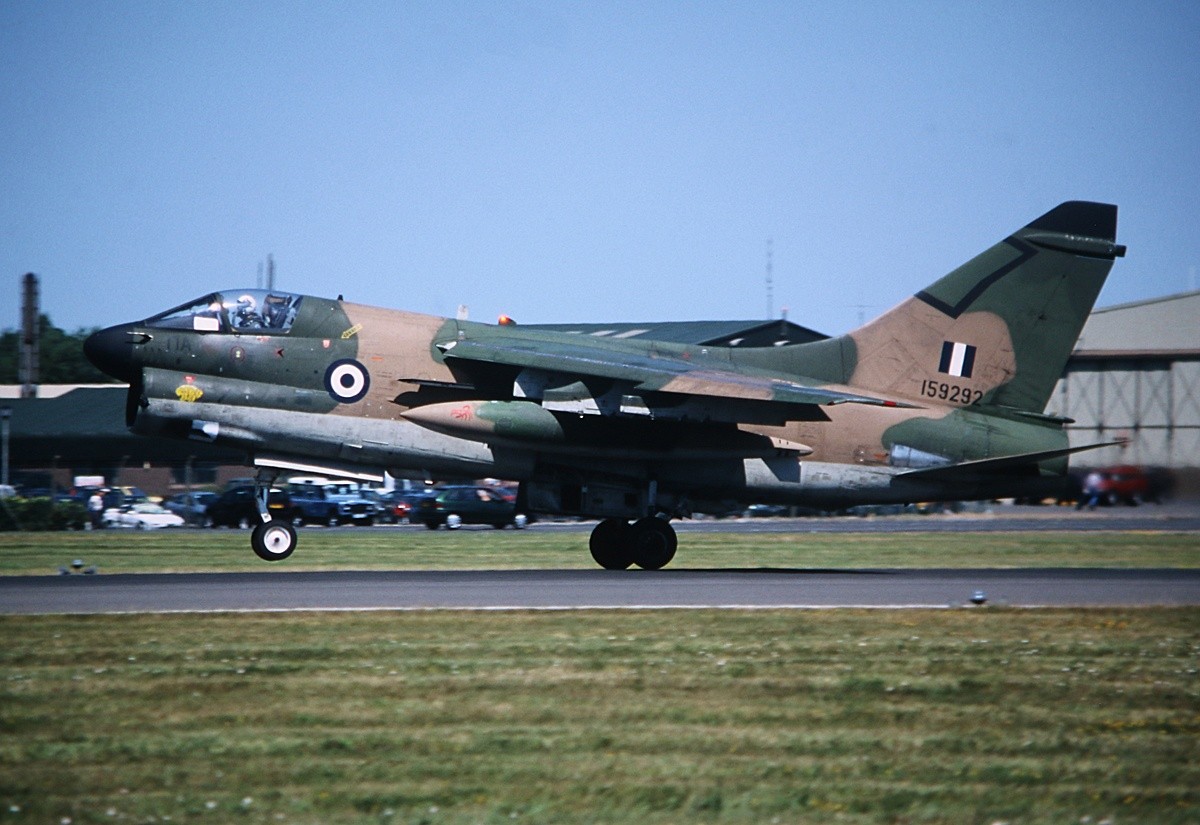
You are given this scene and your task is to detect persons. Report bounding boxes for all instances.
[88,491,105,530]
[1083,472,1101,511]
[237,295,264,329]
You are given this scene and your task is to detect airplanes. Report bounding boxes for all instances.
[83,200,1128,574]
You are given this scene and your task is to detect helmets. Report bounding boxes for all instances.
[237,295,257,314]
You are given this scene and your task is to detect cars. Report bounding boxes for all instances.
[407,483,520,534]
[8,486,187,535]
[155,493,217,529]
[220,476,414,528]
[1079,463,1154,510]
